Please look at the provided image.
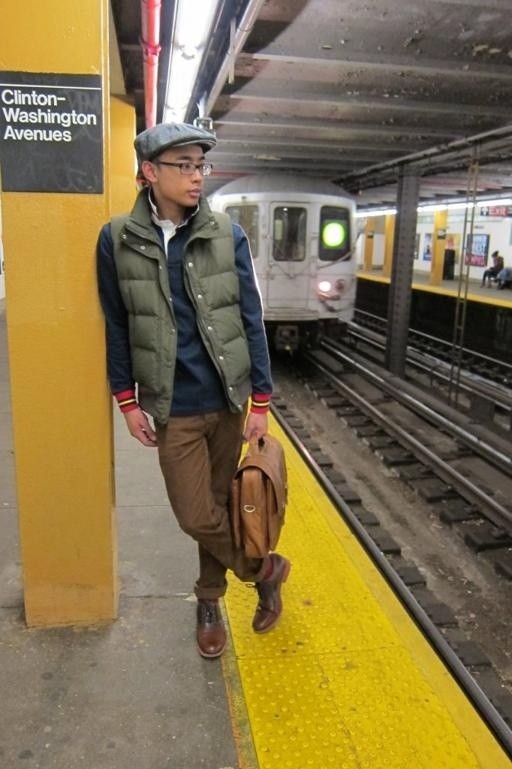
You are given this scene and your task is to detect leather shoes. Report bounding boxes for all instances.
[197,600,227,658]
[253,554,290,633]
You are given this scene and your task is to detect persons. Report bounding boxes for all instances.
[95,121,294,659]
[480,252,503,288]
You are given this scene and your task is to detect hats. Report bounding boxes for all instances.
[134,123,216,170]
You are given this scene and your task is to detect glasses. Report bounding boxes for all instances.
[155,160,212,176]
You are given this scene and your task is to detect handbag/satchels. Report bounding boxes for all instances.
[232,434,287,560]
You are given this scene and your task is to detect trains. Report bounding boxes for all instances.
[206,167,360,360]
